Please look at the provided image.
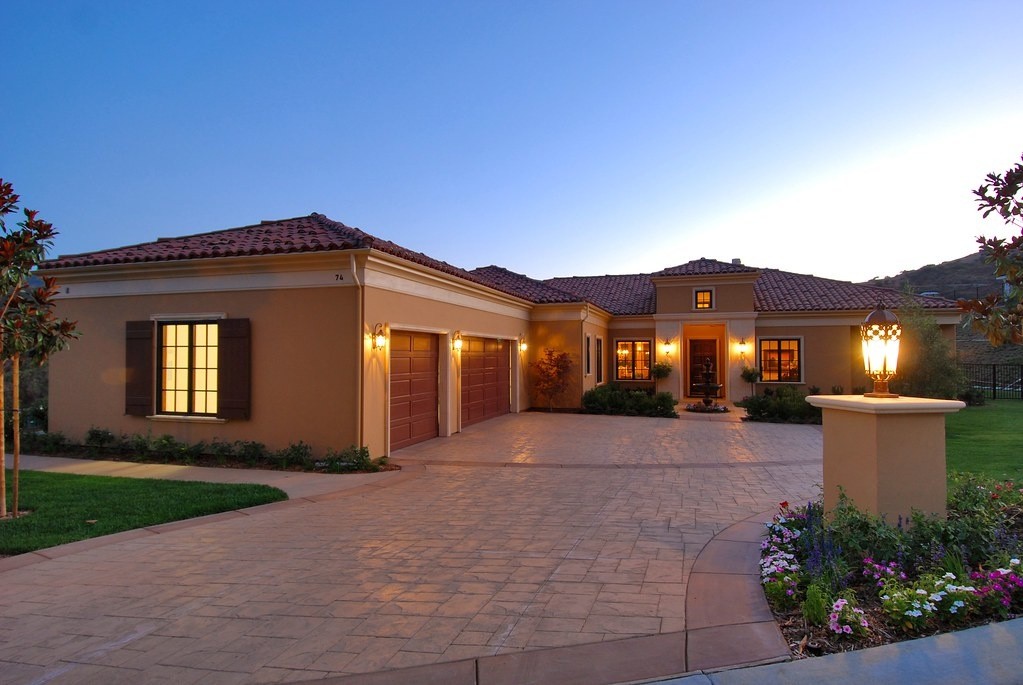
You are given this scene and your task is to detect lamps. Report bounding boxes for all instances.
[452,329,463,351]
[739,337,746,354]
[858,298,902,400]
[371,322,385,350]
[664,339,670,354]
[519,332,527,352]
